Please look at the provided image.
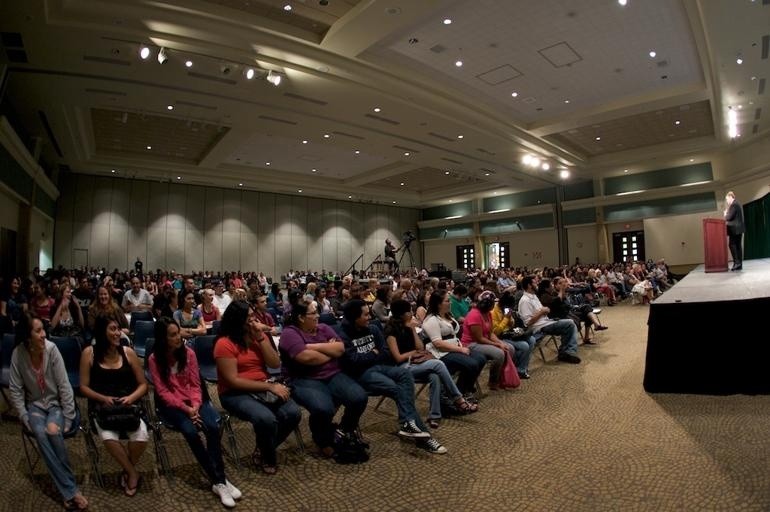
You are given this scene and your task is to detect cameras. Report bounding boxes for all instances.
[504,307,509,315]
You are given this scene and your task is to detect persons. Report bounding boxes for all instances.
[384,240,401,269]
[724,192,745,270]
[0,257,537,509]
[473,255,681,365]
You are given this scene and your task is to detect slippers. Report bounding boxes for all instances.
[121,475,144,497]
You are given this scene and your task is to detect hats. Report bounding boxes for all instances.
[478,290,496,301]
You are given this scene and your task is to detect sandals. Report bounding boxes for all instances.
[253,447,277,474]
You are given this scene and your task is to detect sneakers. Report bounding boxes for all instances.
[399,420,430,437]
[427,438,447,454]
[558,351,581,364]
[583,336,597,345]
[213,479,241,507]
[427,418,440,427]
[594,324,608,330]
[455,398,478,412]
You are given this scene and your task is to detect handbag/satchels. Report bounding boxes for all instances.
[503,327,533,341]
[411,350,432,364]
[429,390,469,416]
[328,423,369,464]
[95,404,142,431]
[250,376,291,403]
[501,352,520,388]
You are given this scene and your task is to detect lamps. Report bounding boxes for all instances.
[137,44,281,87]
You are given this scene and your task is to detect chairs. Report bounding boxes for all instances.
[0,269,670,500]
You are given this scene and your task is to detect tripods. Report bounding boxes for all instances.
[396,241,416,268]
